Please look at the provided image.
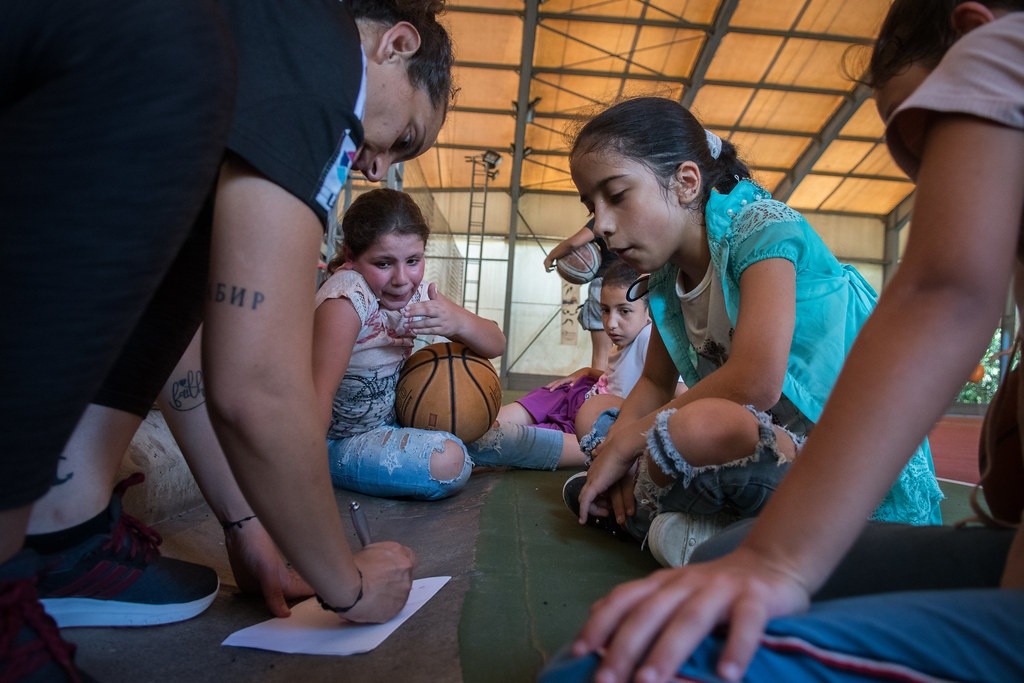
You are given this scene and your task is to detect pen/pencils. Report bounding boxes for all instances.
[348,501,372,547]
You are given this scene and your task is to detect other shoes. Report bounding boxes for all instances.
[648,511,736,568]
[562,471,642,543]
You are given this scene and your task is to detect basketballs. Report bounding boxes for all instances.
[395,341,503,447]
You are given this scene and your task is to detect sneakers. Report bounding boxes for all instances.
[33,472,220,628]
[0,577,99,683]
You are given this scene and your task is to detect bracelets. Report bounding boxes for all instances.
[224,515,255,529]
[314,569,363,613]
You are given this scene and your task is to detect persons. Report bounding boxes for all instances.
[532,0,1024,683]
[0,0,586,683]
[498,97,945,571]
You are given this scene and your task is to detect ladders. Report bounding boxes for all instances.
[462,161,490,316]
